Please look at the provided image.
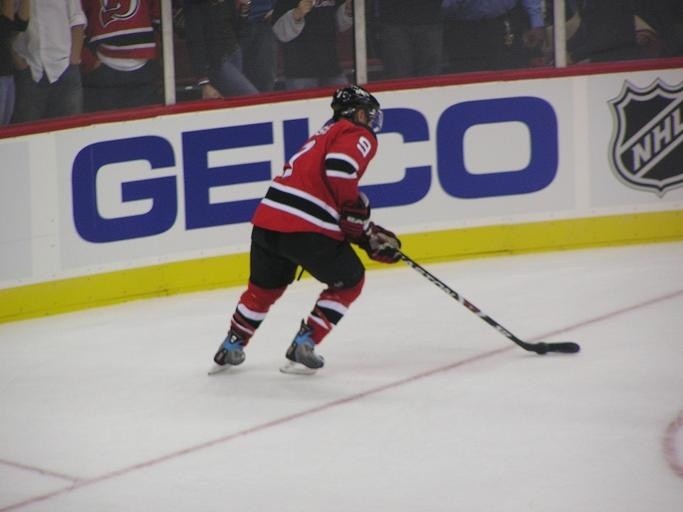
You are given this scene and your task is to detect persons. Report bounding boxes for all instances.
[0,1,683,125]
[212,85,404,368]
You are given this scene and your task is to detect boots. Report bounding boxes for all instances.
[286,318,323,368]
[213,332,245,365]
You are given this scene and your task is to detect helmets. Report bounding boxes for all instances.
[331,85,383,132]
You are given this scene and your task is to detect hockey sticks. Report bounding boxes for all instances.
[396,249,579,354]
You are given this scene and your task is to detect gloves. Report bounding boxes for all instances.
[357,221,402,263]
[339,191,372,239]
